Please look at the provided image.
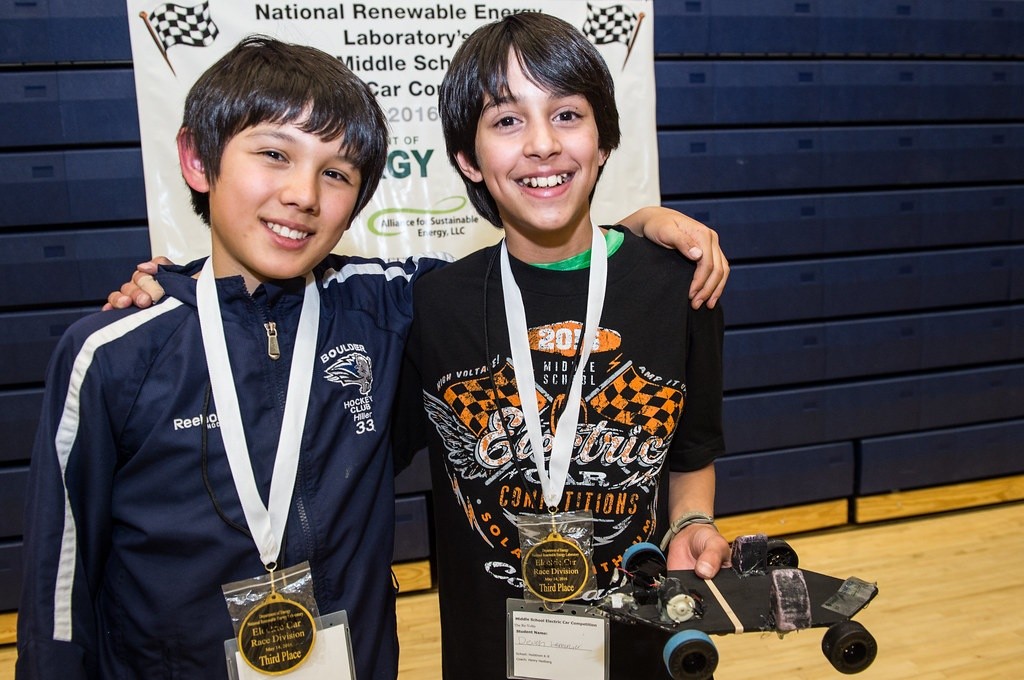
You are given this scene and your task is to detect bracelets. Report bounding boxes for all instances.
[660,509,719,553]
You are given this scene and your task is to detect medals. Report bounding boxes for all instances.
[522,532,589,602]
[238,589,317,678]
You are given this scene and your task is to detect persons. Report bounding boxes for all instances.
[17,33,731,680]
[102,13,724,679]
